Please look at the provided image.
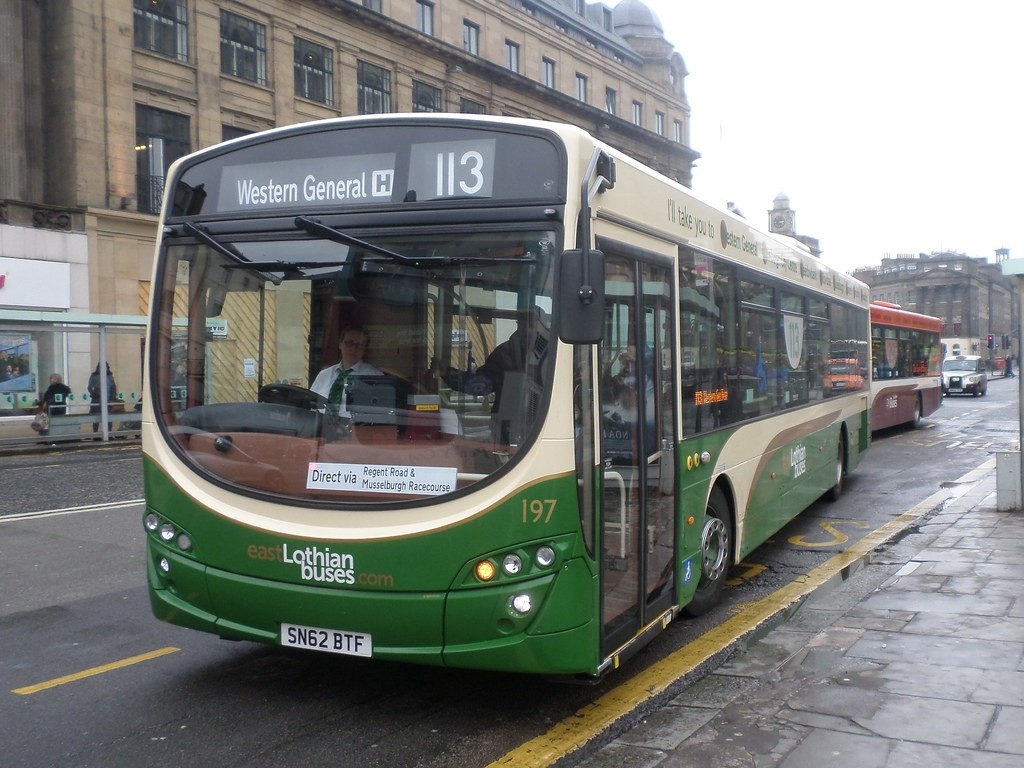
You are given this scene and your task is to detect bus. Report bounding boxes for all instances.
[142,112,873,685]
[870,300,943,431]
[822,359,868,398]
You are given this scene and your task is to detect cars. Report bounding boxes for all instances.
[942,355,988,397]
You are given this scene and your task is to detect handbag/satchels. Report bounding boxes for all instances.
[31,409,49,431]
[109,398,125,412]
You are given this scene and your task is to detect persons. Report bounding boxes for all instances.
[0,350,24,381]
[430,305,551,443]
[87,361,116,440]
[710,353,743,426]
[37,373,72,416]
[310,326,384,419]
[1004,355,1015,377]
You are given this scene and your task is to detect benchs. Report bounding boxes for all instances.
[40,395,143,445]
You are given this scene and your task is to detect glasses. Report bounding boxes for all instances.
[340,340,366,350]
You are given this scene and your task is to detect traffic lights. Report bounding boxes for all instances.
[988,334,995,349]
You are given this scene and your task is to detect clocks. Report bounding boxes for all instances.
[774,216,785,228]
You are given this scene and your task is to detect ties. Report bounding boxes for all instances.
[325,368,353,425]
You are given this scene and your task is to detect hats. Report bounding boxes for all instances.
[619,346,637,362]
